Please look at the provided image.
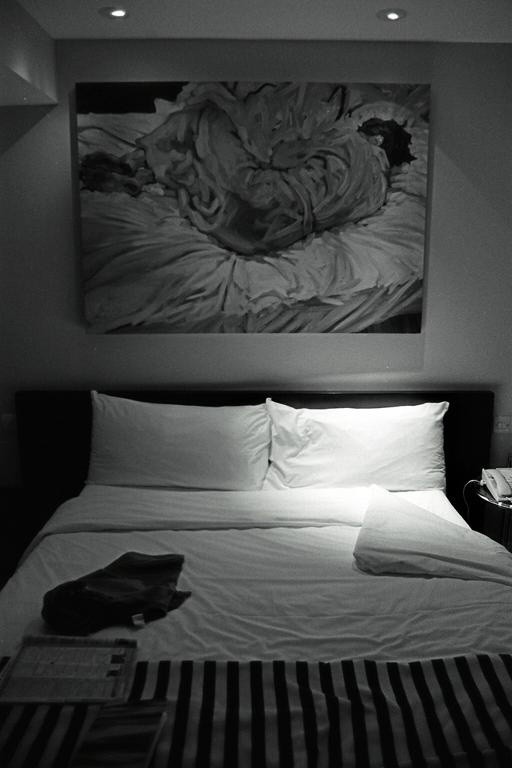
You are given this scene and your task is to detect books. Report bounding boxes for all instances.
[0,634,168,768]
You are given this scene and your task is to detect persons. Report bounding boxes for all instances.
[78,100,417,255]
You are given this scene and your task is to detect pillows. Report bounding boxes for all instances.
[85,389,270,492]
[264,396,449,494]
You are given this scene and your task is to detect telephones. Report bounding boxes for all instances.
[481,467,512,502]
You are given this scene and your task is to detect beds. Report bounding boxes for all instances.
[1,383,512,767]
